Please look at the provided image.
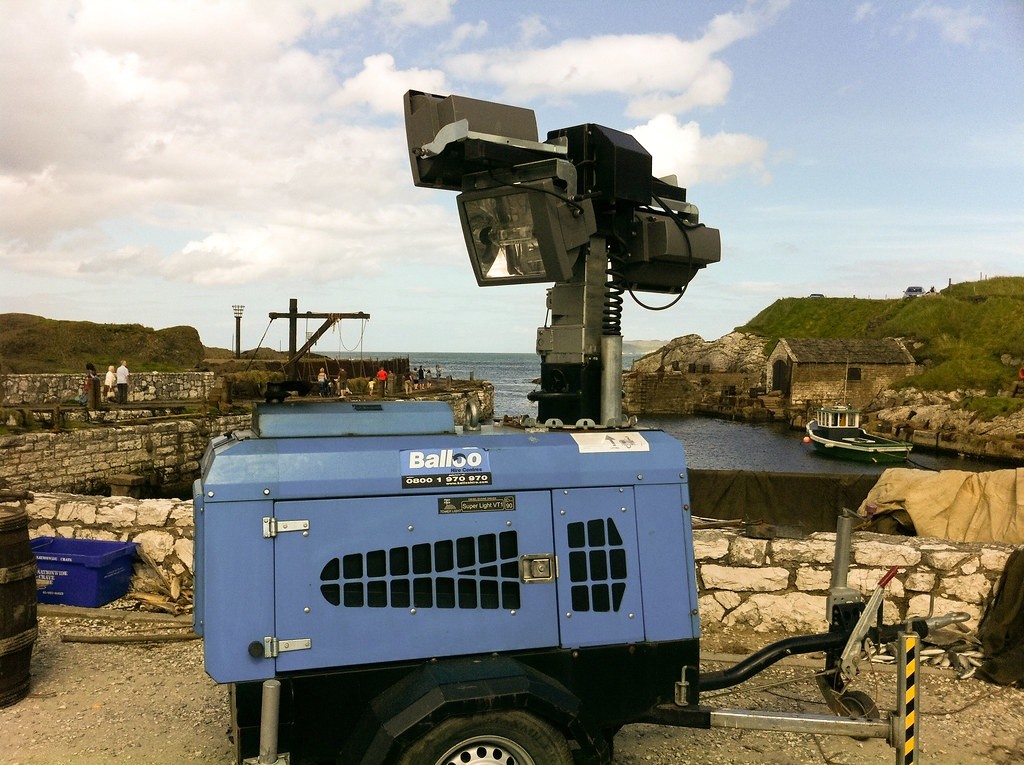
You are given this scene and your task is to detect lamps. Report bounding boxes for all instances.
[403,89,721,310]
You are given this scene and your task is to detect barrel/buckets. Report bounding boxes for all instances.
[0,507,38,710]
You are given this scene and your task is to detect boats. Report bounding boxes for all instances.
[803,404,914,465]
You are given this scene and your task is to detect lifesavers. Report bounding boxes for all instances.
[1018,367,1024,379]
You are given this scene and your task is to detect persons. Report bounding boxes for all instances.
[318,368,327,398]
[436,365,444,383]
[103,366,116,403]
[116,360,132,404]
[367,367,394,396]
[80,372,98,404]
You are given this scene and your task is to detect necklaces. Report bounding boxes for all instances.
[338,366,352,399]
[403,365,431,395]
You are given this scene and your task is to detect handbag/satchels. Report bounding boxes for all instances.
[107,388,116,397]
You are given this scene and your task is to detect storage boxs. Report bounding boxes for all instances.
[30,535,136,608]
